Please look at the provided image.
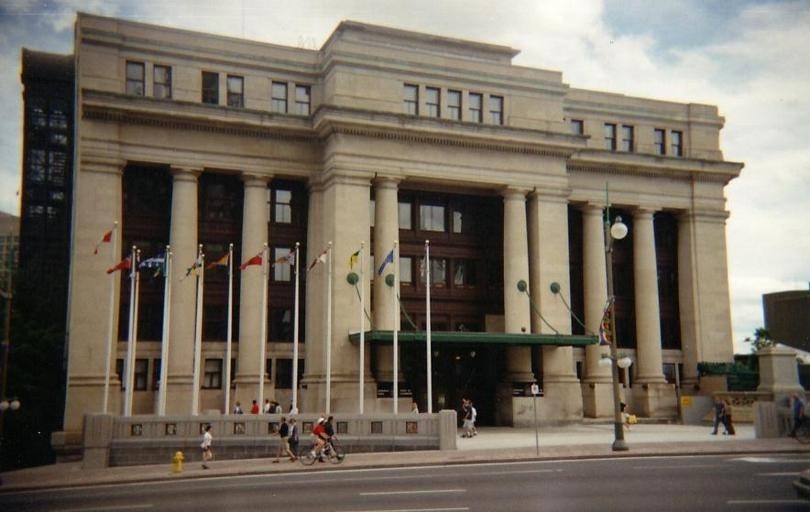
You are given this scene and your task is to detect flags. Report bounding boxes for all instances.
[348,246,361,271]
[378,249,395,276]
[204,253,229,270]
[418,250,427,286]
[240,247,264,270]
[94,228,137,282]
[179,255,202,280]
[138,252,168,272]
[271,249,295,267]
[306,249,328,271]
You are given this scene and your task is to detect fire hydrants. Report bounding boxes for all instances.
[173,451,185,473]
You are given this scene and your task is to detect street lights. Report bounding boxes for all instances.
[597,181,633,452]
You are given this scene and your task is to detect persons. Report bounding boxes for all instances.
[411,403,419,414]
[712,396,730,434]
[199,425,212,469]
[723,399,735,434]
[462,396,478,438]
[252,398,339,463]
[785,390,810,438]
[620,399,632,432]
[234,401,246,434]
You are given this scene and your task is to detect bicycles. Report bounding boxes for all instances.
[795,418,810,445]
[299,437,345,465]
